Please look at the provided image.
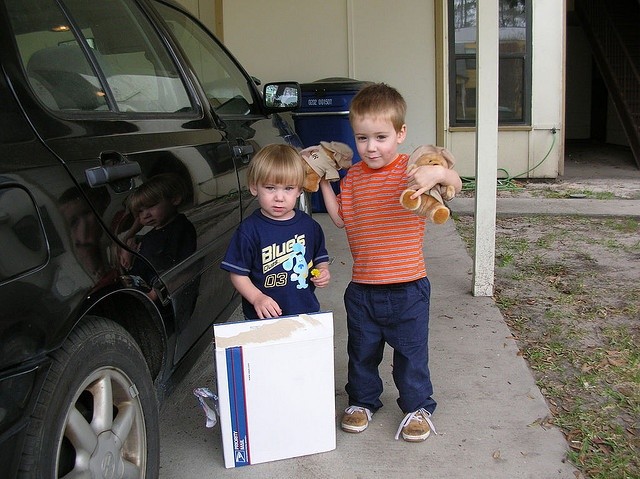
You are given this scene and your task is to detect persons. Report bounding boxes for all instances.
[129,173,201,335]
[220,143,331,321]
[319,81,462,443]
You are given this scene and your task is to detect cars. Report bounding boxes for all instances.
[0,1,312,478]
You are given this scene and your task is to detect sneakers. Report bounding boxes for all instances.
[341,404,373,433]
[394,409,436,442]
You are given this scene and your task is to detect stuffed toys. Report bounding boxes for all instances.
[400,143,456,225]
[298,139,354,193]
